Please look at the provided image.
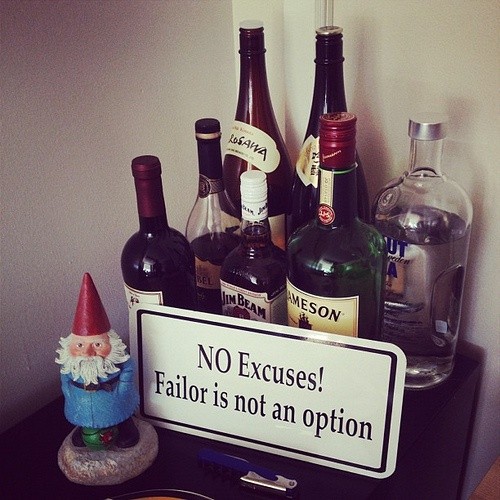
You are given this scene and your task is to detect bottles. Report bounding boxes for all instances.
[120,155,197,312]
[371,112,473,389]
[289,26,371,234]
[222,18,292,255]
[287,112,387,342]
[186,118,242,316]
[220,170,287,325]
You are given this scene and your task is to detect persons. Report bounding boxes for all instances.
[53,272,140,450]
